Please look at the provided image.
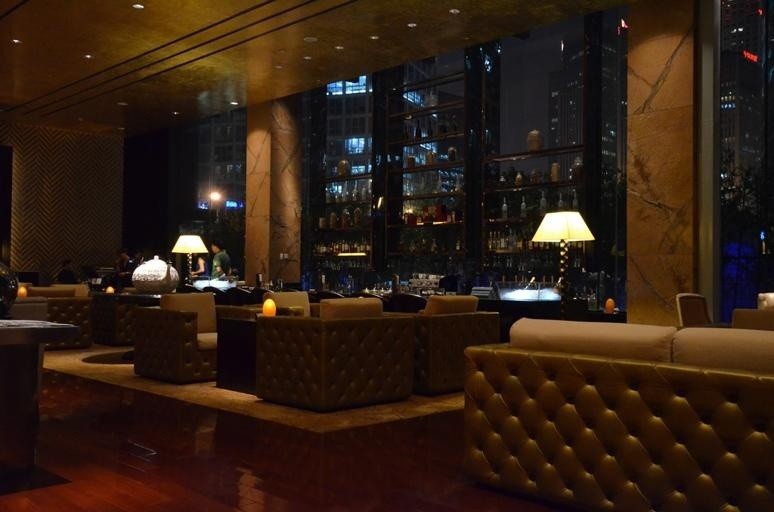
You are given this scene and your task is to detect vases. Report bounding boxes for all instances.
[319,126,585,230]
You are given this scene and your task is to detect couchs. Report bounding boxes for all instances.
[134,266,500,412]
[11,268,162,348]
[462,317,774,512]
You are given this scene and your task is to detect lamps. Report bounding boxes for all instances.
[531,211,596,321]
[170,234,209,286]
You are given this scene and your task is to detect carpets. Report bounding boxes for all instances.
[0,463,72,497]
[41,346,464,435]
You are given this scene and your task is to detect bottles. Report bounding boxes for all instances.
[501,191,579,219]
[314,236,372,254]
[498,157,582,188]
[526,129,546,152]
[319,208,362,231]
[255,270,401,298]
[553,278,562,295]
[338,159,350,176]
[487,231,583,249]
[522,276,536,289]
[403,113,460,166]
[326,180,372,202]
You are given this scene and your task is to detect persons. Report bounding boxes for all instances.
[60,258,88,283]
[115,237,233,282]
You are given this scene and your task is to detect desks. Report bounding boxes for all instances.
[0,318,81,475]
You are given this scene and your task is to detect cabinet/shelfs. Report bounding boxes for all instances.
[298,7,627,290]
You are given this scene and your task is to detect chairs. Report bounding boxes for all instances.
[731,305,774,330]
[674,291,712,329]
[756,292,774,309]
